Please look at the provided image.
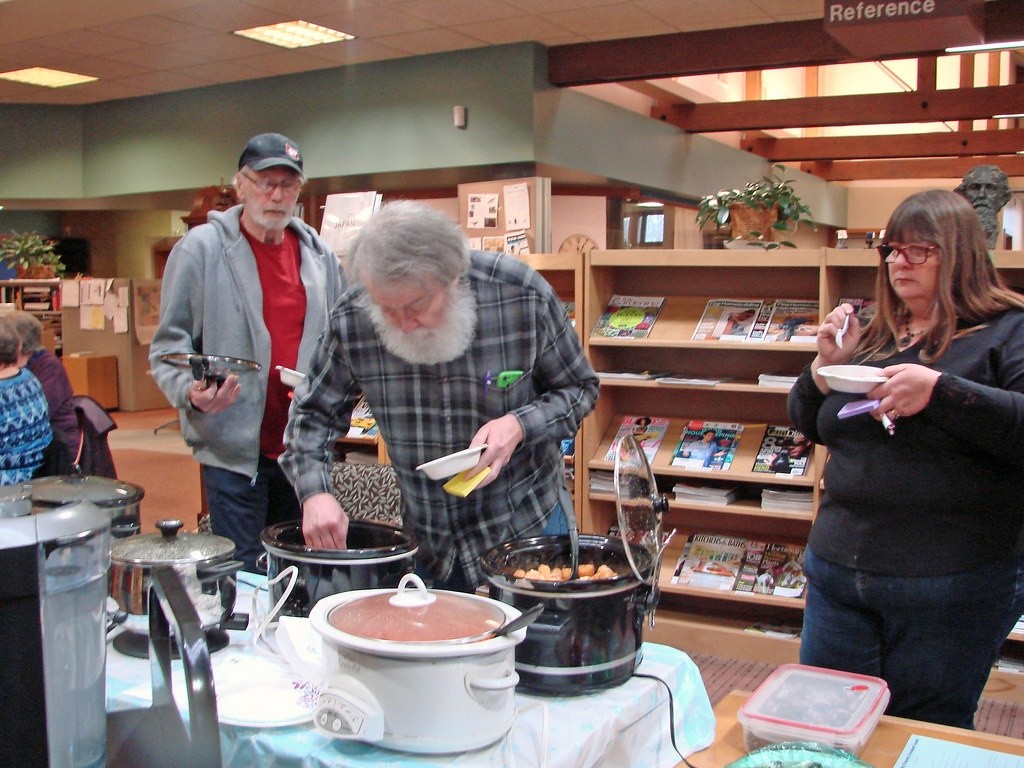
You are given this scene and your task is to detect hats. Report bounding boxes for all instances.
[239,132,304,176]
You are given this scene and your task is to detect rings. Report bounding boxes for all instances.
[890,409,898,417]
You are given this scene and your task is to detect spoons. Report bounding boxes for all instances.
[835,315,850,348]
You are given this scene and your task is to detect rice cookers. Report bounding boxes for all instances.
[14,465,147,542]
[480,432,668,696]
[263,519,418,623]
[309,573,527,755]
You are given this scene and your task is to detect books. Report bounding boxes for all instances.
[1,287,60,311]
[319,190,382,258]
[347,394,380,440]
[558,294,878,638]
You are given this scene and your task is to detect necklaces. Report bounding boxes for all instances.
[898,310,925,347]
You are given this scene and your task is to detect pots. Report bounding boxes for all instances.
[109,519,250,637]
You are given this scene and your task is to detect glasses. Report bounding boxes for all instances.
[877,242,941,265]
[239,169,304,194]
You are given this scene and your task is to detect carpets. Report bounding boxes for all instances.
[680,650,780,707]
[974,698,1024,742]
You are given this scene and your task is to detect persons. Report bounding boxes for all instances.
[277,200,599,589]
[763,430,811,473]
[778,555,802,587]
[953,165,1011,248]
[756,570,773,588]
[787,188,1024,730]
[6,310,80,464]
[773,313,814,342]
[683,430,727,467]
[790,569,806,589]
[631,417,652,436]
[724,309,756,335]
[0,319,54,483]
[148,133,350,577]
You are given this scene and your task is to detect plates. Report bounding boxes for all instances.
[177,663,313,727]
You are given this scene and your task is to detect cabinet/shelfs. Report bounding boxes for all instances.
[346,248,1024,646]
[0,277,171,412]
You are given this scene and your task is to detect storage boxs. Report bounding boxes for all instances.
[737,664,891,758]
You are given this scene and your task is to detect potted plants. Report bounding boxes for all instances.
[0,228,66,279]
[696,164,819,250]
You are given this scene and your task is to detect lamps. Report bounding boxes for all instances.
[945,37,1024,53]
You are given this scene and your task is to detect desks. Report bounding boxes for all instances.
[104,571,1024,768]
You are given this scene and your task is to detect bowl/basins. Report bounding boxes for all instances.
[276,364,306,388]
[816,363,888,394]
[736,662,891,758]
[415,445,487,480]
